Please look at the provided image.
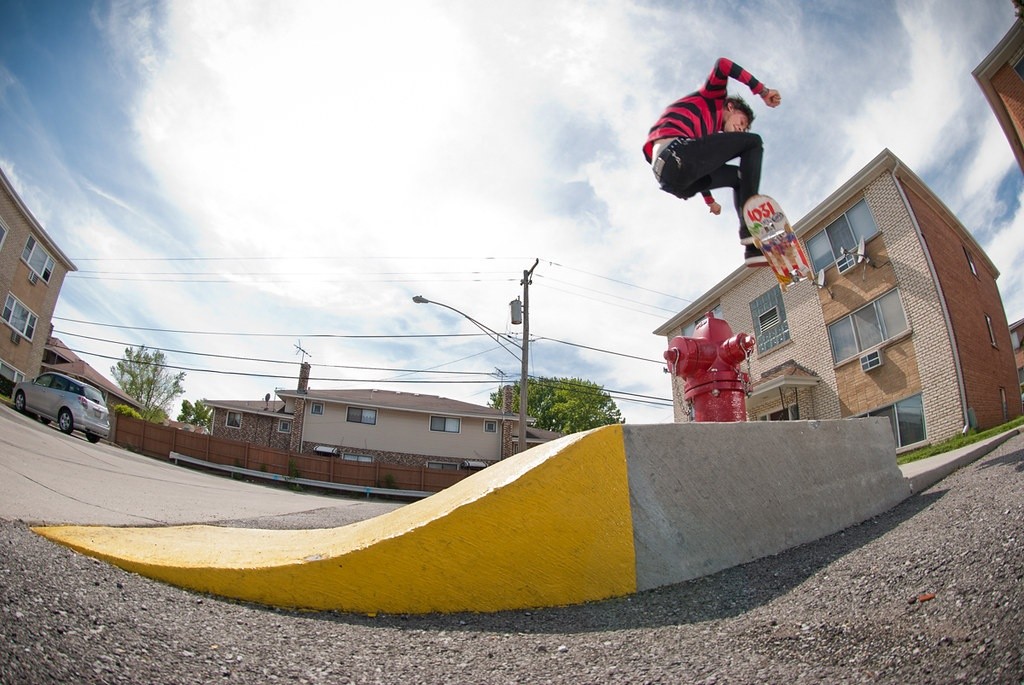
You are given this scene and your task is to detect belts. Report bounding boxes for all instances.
[654,137,687,183]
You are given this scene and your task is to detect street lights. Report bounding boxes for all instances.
[412,296,528,452]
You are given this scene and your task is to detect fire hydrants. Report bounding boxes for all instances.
[662,311,757,423]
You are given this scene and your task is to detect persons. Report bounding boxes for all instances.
[642,58,782,268]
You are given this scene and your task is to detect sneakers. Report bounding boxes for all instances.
[745,250,770,267]
[739,229,753,245]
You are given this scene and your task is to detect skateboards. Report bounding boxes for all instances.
[742,195,817,294]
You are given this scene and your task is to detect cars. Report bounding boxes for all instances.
[12,372,110,443]
